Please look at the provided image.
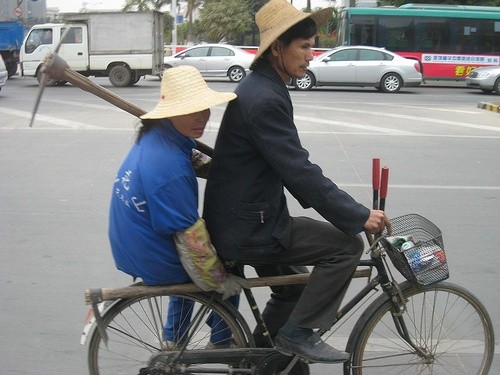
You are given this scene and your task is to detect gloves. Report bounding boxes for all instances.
[217,273,252,301]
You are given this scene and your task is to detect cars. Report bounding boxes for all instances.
[159,42,257,84]
[289,43,423,93]
[466,65,500,97]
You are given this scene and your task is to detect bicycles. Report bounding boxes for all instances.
[79,210,497,375]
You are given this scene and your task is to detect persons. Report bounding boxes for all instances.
[108,65,249,351]
[362,28,373,46]
[423,33,434,49]
[203,0,392,366]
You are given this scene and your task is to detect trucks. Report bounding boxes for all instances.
[15,8,166,88]
[0,20,27,80]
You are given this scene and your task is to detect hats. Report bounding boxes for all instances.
[140,65,237,119]
[249,0,333,71]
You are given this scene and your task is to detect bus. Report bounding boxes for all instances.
[337,2,500,83]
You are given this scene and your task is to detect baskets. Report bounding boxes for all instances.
[379,213,450,289]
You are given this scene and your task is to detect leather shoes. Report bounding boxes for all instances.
[276,329,350,364]
